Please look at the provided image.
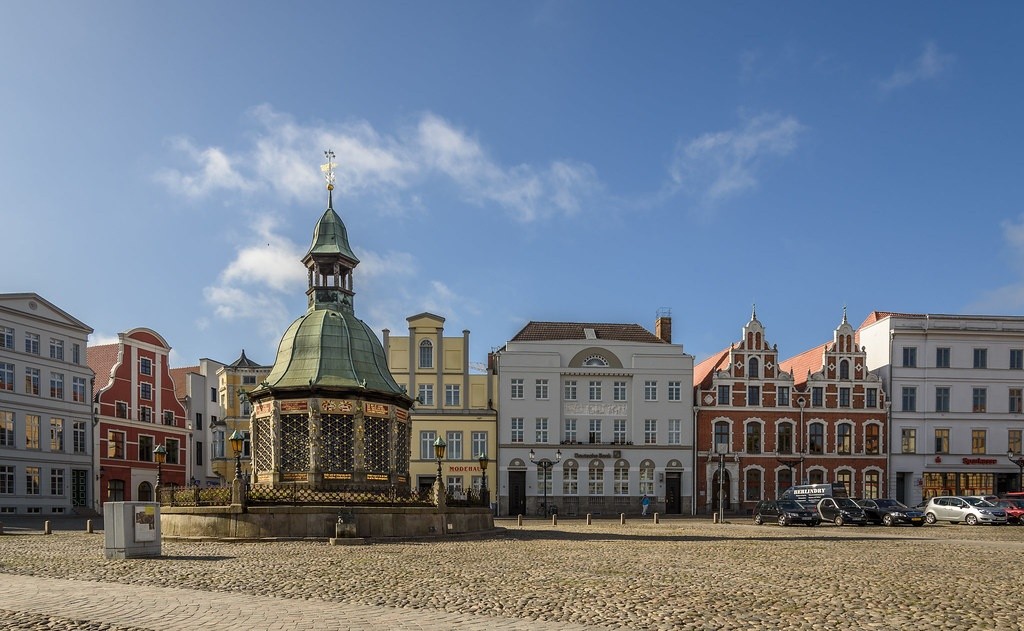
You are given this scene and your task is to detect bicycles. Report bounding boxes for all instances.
[537,503,558,517]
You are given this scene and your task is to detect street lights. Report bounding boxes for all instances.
[528,448,561,519]
[153,443,168,490]
[1007,449,1024,492]
[775,450,806,487]
[227,429,246,506]
[479,452,489,501]
[706,448,741,523]
[433,436,446,506]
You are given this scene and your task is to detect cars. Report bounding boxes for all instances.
[926,496,1008,525]
[754,499,817,526]
[814,495,868,526]
[856,498,926,527]
[911,500,930,512]
[988,498,1024,525]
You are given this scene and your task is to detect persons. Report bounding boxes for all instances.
[641,494,650,516]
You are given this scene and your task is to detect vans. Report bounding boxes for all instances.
[779,483,849,503]
